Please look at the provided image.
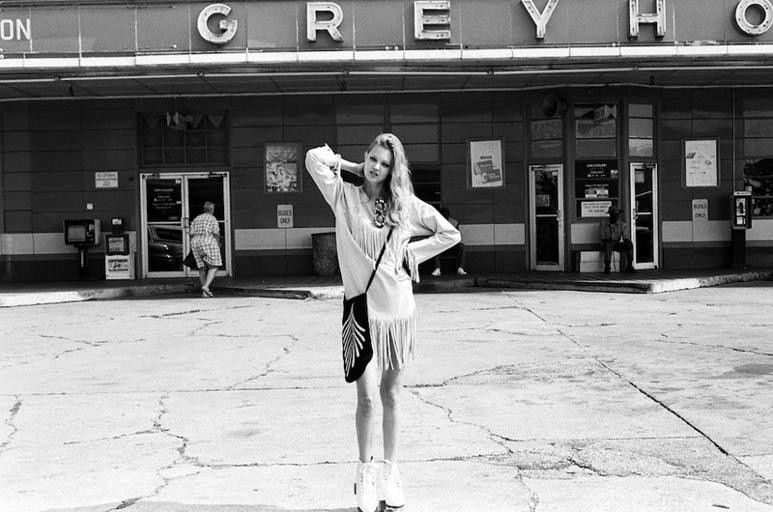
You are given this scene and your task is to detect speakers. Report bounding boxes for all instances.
[351,453,406,512]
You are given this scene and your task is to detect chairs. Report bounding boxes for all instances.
[200,286,215,298]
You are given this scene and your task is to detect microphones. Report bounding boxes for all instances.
[572,250,622,273]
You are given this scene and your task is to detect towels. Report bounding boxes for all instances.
[311,232,339,276]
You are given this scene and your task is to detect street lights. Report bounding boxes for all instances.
[181,250,200,271]
[339,291,374,384]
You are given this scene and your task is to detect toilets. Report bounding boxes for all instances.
[729,194,752,230]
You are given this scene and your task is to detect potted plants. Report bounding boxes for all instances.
[465,134,506,191]
[261,141,305,195]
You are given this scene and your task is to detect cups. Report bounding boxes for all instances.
[373,197,388,228]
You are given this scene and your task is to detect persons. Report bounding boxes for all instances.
[188,202,225,299]
[303,130,463,511]
[430,207,468,276]
[599,204,635,273]
